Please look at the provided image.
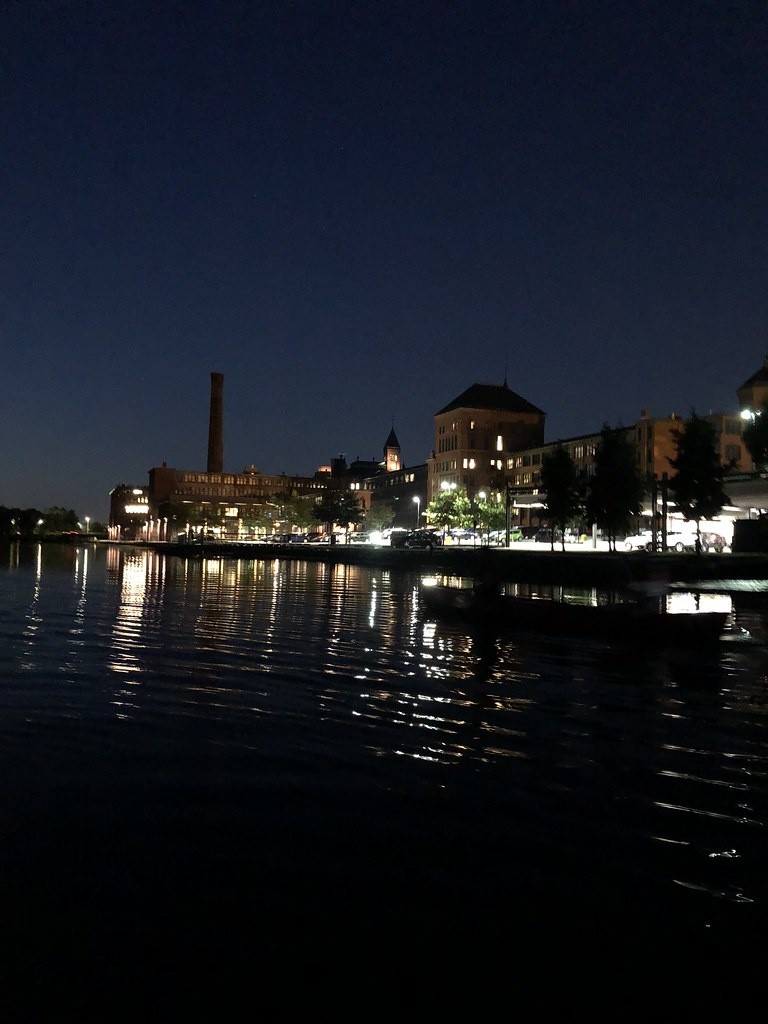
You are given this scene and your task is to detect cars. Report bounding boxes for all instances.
[450,525,563,546]
[264,531,371,544]
[624,531,727,554]
[391,530,442,549]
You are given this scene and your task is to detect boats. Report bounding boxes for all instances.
[419,555,737,644]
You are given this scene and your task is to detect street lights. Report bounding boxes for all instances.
[478,492,487,502]
[85,516,90,533]
[412,496,420,529]
